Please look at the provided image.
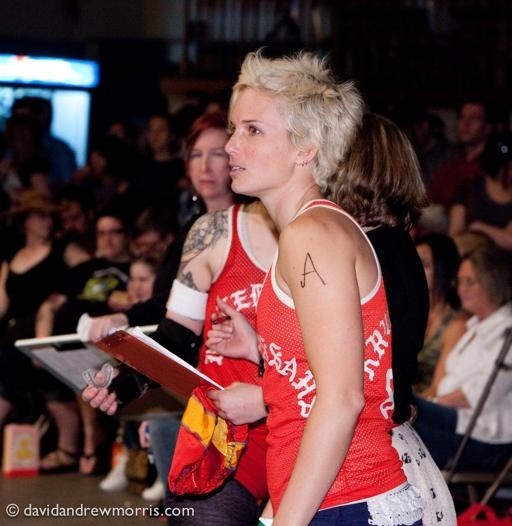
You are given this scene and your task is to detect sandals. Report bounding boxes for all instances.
[40,446,78,474]
[77,452,98,475]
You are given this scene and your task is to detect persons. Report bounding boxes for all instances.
[1,2,511,526]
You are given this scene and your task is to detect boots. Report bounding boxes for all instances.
[141,476,165,501]
[98,452,129,492]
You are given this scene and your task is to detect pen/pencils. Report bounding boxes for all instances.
[213,316,230,324]
